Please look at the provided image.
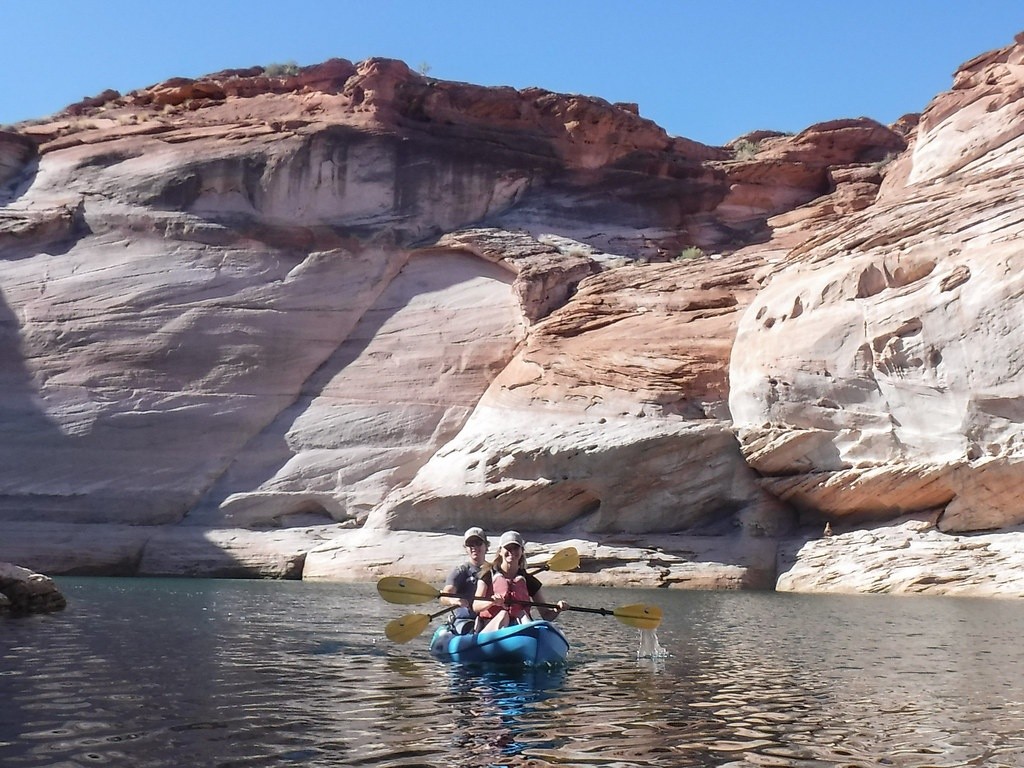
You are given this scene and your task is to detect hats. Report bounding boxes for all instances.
[499,531,523,548]
[464,527,487,545]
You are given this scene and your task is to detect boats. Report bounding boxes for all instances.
[429,621,571,668]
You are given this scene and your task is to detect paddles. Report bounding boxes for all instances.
[385,546,581,645]
[377,575,663,628]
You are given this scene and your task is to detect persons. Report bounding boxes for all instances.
[473,530,569,634]
[439,527,491,635]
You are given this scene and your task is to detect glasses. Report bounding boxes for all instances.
[466,540,483,546]
